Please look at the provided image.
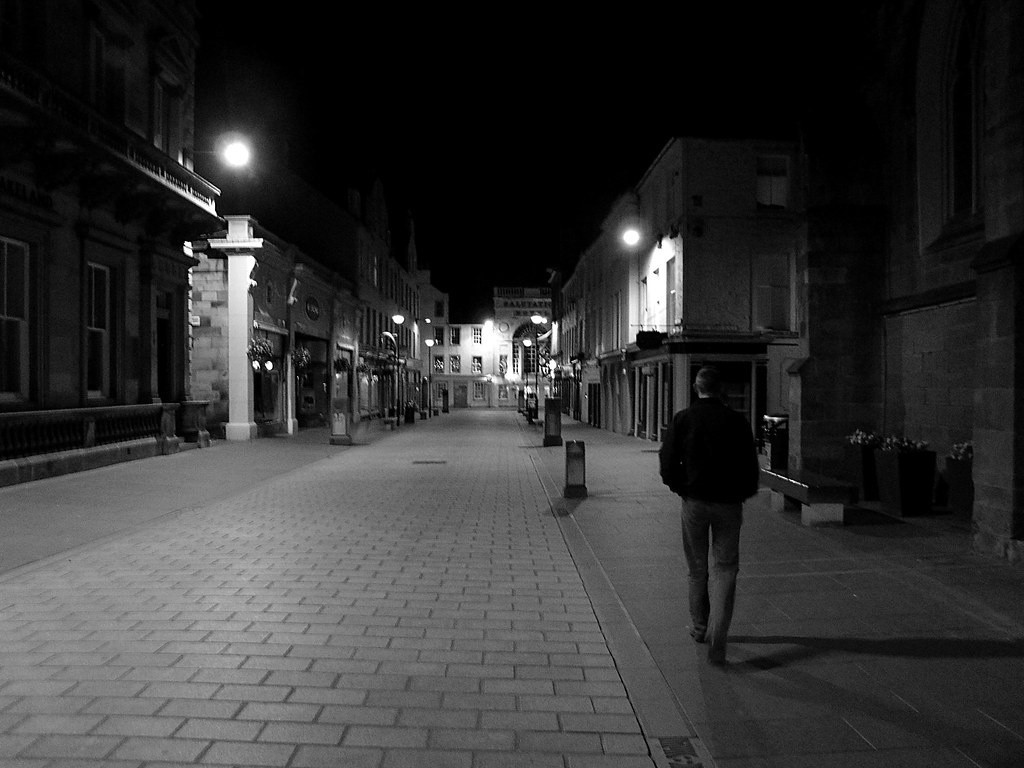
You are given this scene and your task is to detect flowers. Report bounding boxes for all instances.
[882,435,930,451]
[847,428,875,446]
[952,440,974,461]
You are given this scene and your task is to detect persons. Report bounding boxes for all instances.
[659,366,759,664]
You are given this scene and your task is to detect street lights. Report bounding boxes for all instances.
[529,312,543,393]
[425,337,435,419]
[391,313,406,427]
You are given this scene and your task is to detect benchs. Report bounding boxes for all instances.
[759,466,843,526]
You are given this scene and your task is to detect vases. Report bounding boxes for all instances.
[942,464,974,520]
[837,445,880,502]
[872,449,937,516]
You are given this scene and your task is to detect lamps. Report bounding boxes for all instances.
[415,317,431,324]
[670,218,683,239]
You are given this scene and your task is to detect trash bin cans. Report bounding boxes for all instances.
[762,413,789,477]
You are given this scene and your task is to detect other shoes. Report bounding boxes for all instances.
[690,628,705,643]
[707,650,727,667]
